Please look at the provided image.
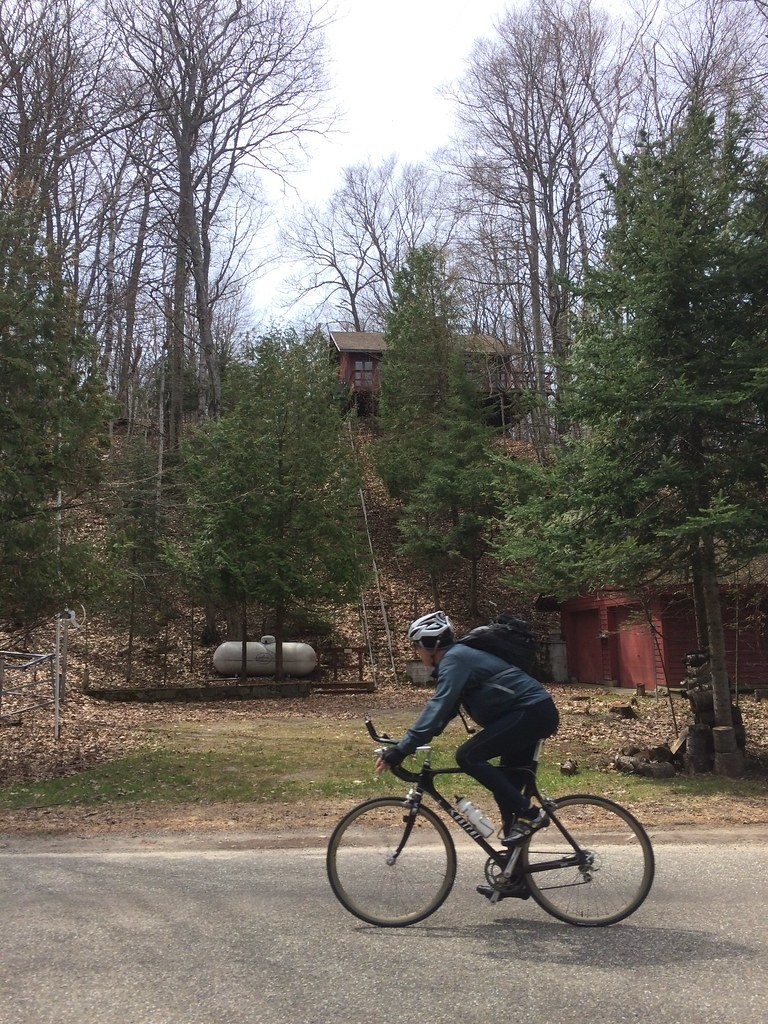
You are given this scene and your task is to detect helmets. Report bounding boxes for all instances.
[407,610,454,650]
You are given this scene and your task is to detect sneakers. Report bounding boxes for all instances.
[476,883,531,899]
[502,808,550,845]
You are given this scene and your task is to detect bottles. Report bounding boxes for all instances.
[453,794,494,839]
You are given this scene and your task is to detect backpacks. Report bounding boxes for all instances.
[430,622,554,684]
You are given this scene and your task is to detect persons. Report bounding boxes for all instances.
[374,612,560,899]
